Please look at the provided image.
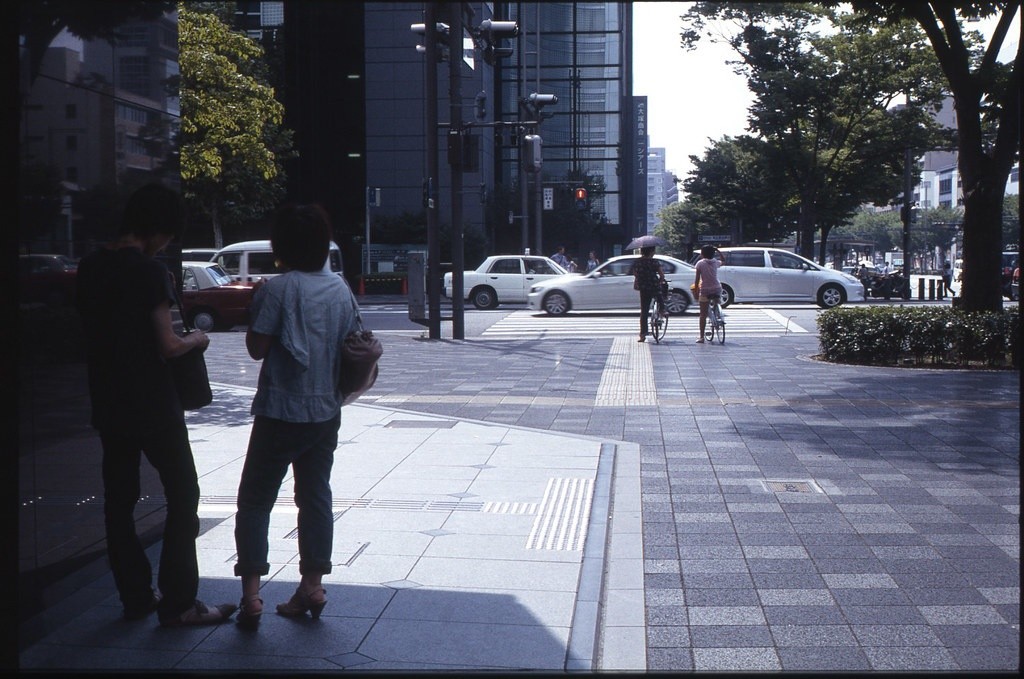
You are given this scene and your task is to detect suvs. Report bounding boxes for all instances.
[687,246,865,310]
[1001,251,1020,301]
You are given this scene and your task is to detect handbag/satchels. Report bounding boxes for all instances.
[658,278,668,300]
[167,273,213,411]
[338,273,383,405]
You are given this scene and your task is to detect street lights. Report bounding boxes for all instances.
[901,16,981,301]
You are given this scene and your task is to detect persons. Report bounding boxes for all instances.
[234,203,362,626]
[528,261,539,274]
[584,250,599,272]
[694,245,726,342]
[852,261,891,296]
[627,245,669,343]
[549,246,578,273]
[941,261,954,297]
[76,183,237,628]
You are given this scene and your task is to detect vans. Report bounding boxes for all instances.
[208,238,345,284]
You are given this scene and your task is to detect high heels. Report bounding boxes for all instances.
[276,582,328,621]
[236,594,264,632]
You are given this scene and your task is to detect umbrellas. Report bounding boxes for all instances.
[625,235,669,248]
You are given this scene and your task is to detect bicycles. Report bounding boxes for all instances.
[634,277,674,345]
[691,280,727,345]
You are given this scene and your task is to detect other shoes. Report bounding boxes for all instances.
[123,587,162,620]
[953,292,955,296]
[158,600,238,627]
[696,337,704,343]
[638,338,646,342]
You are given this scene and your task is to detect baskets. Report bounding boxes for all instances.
[659,284,673,302]
[690,288,701,299]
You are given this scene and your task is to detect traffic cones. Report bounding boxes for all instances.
[399,278,409,296]
[358,278,366,296]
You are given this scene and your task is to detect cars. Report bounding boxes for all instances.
[952,259,963,282]
[18,255,77,302]
[179,281,258,333]
[841,264,880,277]
[182,261,233,292]
[527,254,702,317]
[440,255,584,311]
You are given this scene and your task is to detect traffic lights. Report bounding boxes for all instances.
[910,206,922,224]
[415,44,449,63]
[484,45,515,65]
[538,109,555,122]
[480,18,520,44]
[411,21,450,42]
[575,188,587,211]
[529,92,559,110]
